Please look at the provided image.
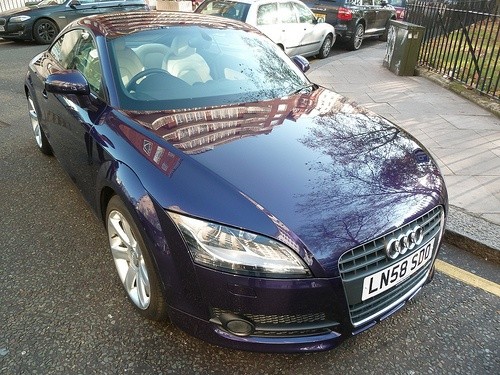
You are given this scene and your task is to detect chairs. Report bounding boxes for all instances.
[135,43,169,73]
[162,34,212,86]
[112,37,144,89]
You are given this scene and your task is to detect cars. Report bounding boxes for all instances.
[301,0,447,51]
[24,11,449,353]
[193,0,336,60]
[0,0,152,46]
